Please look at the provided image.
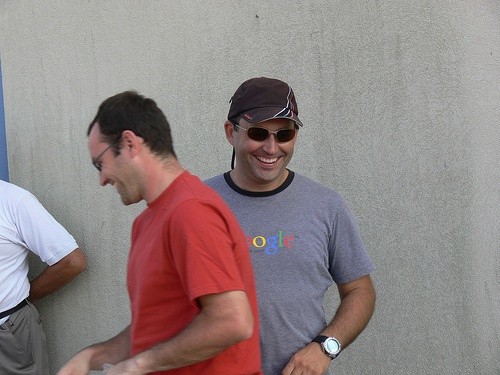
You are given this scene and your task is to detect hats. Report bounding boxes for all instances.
[227,77,303,126]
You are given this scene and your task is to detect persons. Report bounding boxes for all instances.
[0,178,87,375]
[201,77,378,375]
[58,91,262,375]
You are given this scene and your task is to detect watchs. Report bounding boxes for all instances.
[312,335,342,360]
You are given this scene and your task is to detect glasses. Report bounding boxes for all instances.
[235,124,297,144]
[92,134,122,172]
